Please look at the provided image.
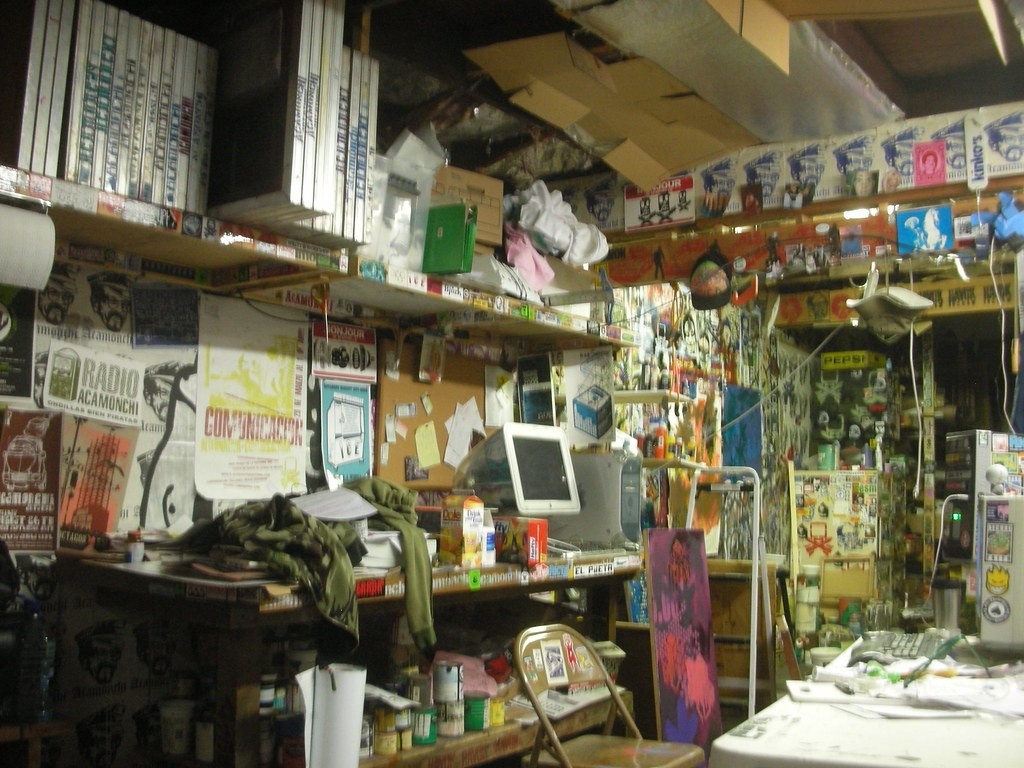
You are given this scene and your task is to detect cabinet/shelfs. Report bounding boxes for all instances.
[0,164,635,768]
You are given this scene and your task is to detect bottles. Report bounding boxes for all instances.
[17,612,55,722]
[653,420,666,459]
[860,443,872,471]
[193,703,218,765]
[663,430,674,460]
[124,530,146,564]
[642,434,653,458]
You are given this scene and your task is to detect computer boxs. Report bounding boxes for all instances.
[941,429,1024,562]
[544,455,643,549]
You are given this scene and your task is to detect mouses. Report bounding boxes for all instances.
[847,651,894,666]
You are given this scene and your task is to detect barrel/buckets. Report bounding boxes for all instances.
[794,602,819,634]
[865,598,893,632]
[816,630,856,650]
[810,647,842,668]
[839,596,863,624]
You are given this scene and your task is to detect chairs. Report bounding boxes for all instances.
[516,626,705,768]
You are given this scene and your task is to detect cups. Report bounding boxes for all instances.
[931,577,961,632]
[817,445,834,470]
[765,553,787,568]
[801,564,821,576]
[159,698,194,756]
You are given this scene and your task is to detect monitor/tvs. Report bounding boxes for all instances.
[451,421,581,517]
[976,495,1024,652]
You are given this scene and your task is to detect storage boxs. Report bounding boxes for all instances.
[566,638,627,695]
[430,165,506,246]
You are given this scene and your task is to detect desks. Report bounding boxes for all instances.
[709,633,1024,768]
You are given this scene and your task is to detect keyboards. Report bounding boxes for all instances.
[876,632,935,659]
